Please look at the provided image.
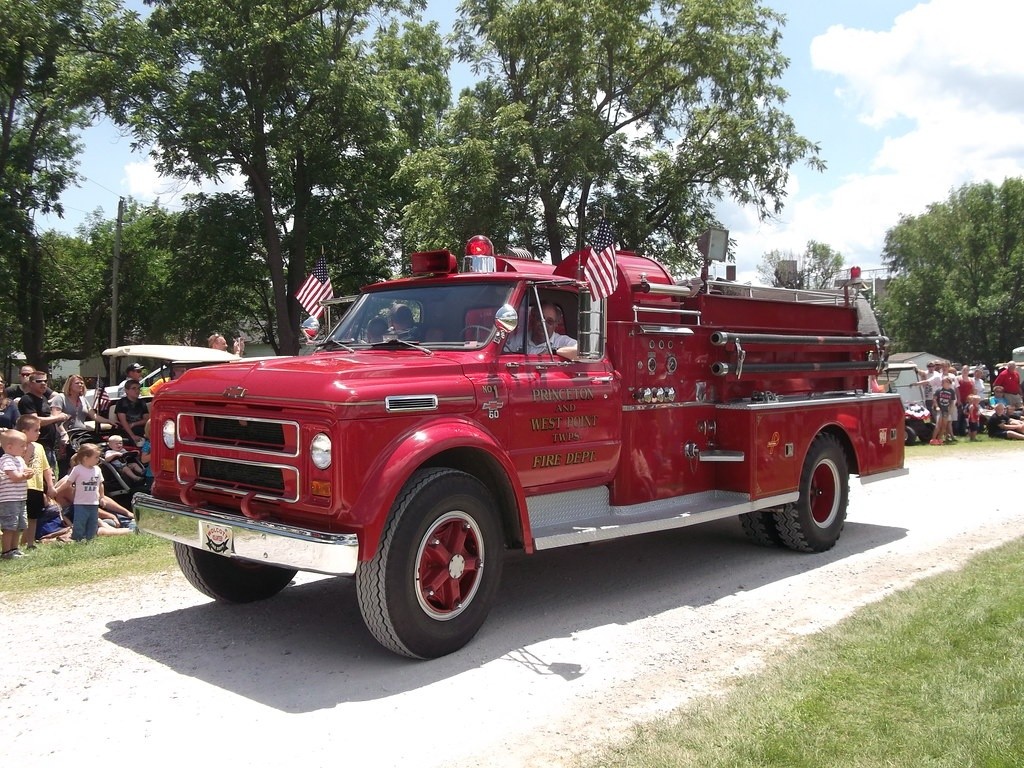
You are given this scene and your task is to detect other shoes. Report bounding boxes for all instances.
[14,550,25,555]
[930,438,938,444]
[937,439,944,444]
[946,433,952,441]
[2,551,20,559]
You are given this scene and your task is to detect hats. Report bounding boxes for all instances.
[935,360,941,364]
[928,362,935,365]
[126,362,145,372]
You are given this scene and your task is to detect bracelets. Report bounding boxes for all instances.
[551,346,561,355]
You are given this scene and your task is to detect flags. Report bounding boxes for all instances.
[93,374,110,411]
[294,253,334,320]
[583,215,619,302]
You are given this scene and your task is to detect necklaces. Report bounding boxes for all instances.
[69,395,79,415]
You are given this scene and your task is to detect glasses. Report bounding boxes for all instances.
[135,369,142,373]
[32,379,48,383]
[547,317,556,325]
[214,334,219,341]
[131,387,140,390]
[21,373,30,377]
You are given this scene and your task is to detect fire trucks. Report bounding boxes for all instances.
[135,234,908,662]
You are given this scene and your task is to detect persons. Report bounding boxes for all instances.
[386,304,420,339]
[503,303,579,360]
[302,316,320,329]
[149,366,185,393]
[207,333,241,357]
[366,319,389,343]
[871,358,1024,444]
[0,362,156,550]
[0,428,35,561]
